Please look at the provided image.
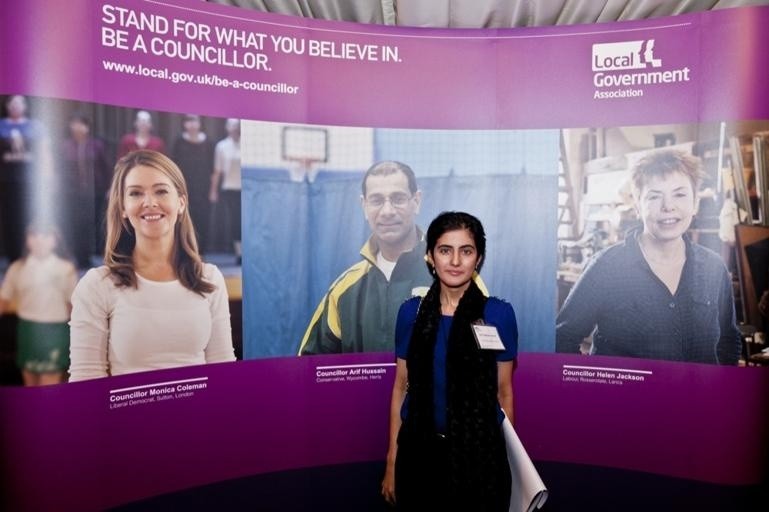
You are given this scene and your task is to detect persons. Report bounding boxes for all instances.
[118,110,241,266]
[295,159,490,359]
[0,93,114,269]
[376,209,522,511]
[553,147,744,366]
[67,149,237,383]
[0,216,79,387]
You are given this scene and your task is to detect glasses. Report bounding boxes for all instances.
[365,193,417,207]
